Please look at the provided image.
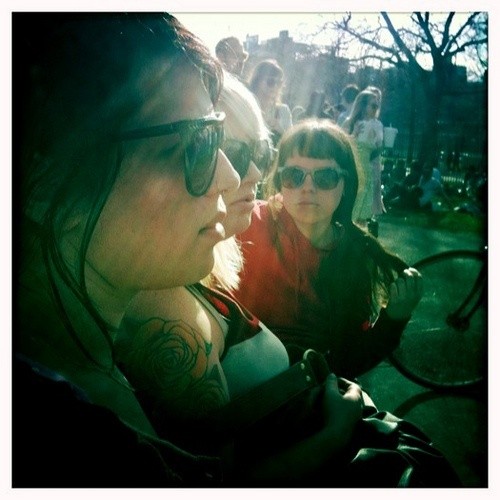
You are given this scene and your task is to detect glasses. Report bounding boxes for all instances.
[234,50,249,61]
[225,141,278,179]
[276,166,349,191]
[370,103,379,111]
[118,112,227,196]
[267,78,282,89]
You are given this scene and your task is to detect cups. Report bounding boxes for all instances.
[382,127,397,146]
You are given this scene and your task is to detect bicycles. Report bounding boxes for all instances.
[384,167,488,397]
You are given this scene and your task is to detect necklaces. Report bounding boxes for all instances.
[72,357,136,395]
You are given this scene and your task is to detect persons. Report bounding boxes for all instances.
[211,34,484,243]
[197,118,426,379]
[111,67,464,489]
[12,12,266,487]
